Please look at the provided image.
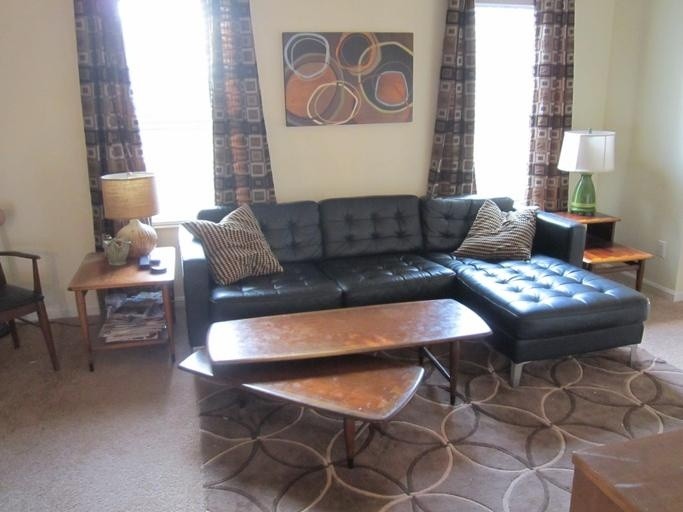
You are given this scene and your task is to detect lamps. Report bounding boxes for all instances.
[557,128,616,216]
[101,172,158,258]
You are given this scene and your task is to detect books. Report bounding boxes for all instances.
[98,290,167,343]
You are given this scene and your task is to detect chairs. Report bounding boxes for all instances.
[0,251,60,370]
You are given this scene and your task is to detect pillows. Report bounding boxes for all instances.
[181,204,283,285]
[451,199,540,262]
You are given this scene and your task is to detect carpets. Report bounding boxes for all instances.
[188,345,683,511]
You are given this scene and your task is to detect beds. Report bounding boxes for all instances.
[420,198,648,386]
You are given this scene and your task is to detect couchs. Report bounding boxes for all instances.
[178,194,455,350]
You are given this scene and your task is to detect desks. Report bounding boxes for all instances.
[552,211,654,292]
[68,246,176,372]
[569,431,683,511]
